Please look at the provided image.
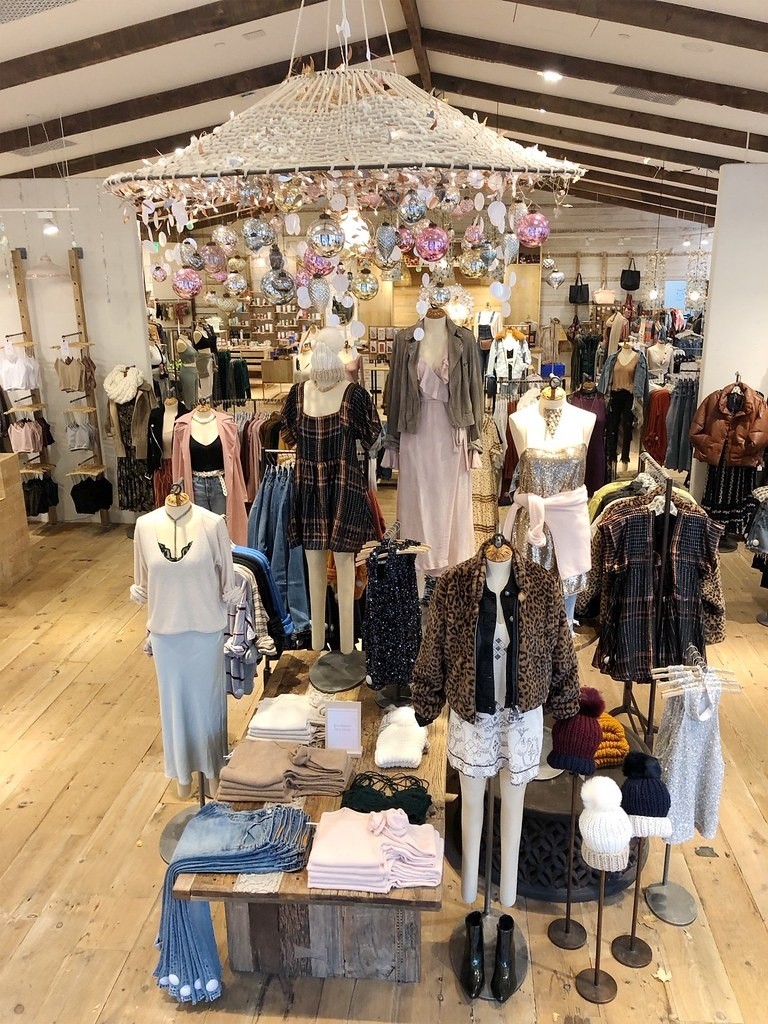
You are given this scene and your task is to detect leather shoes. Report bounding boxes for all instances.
[461,911,485,1000]
[491,914,517,1003]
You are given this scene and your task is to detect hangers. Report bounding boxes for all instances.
[729,374,745,397]
[66,458,108,478]
[541,319,560,330]
[675,323,704,341]
[0,335,36,350]
[208,397,286,412]
[63,398,98,415]
[651,645,740,698]
[266,449,296,477]
[19,460,57,475]
[578,321,600,336]
[636,452,676,513]
[754,486,768,502]
[4,403,48,416]
[659,355,701,392]
[49,334,95,350]
[496,380,551,402]
[355,521,431,568]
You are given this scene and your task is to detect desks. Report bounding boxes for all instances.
[230,345,294,405]
[171,650,450,982]
[365,362,390,405]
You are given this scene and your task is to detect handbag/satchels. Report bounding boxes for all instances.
[620,258,640,291]
[565,315,581,342]
[569,273,589,303]
[622,294,637,318]
[480,339,492,350]
[553,318,567,341]
[159,363,169,379]
[592,283,617,304]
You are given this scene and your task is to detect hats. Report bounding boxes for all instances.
[620,752,673,837]
[578,776,633,872]
[592,713,629,769]
[309,328,345,381]
[546,687,606,777]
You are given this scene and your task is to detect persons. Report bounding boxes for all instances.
[130,483,237,800]
[193,324,215,398]
[383,303,485,572]
[342,348,364,386]
[172,399,249,546]
[146,397,190,508]
[413,533,580,908]
[279,342,383,654]
[292,344,314,385]
[603,312,629,364]
[104,367,157,540]
[176,330,198,408]
[598,343,646,473]
[149,339,166,397]
[647,339,674,375]
[509,377,600,632]
[486,327,530,391]
[567,378,611,502]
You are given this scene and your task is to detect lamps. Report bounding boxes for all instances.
[101,1,587,370]
[37,211,59,235]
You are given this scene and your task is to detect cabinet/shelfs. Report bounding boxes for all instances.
[223,297,327,351]
